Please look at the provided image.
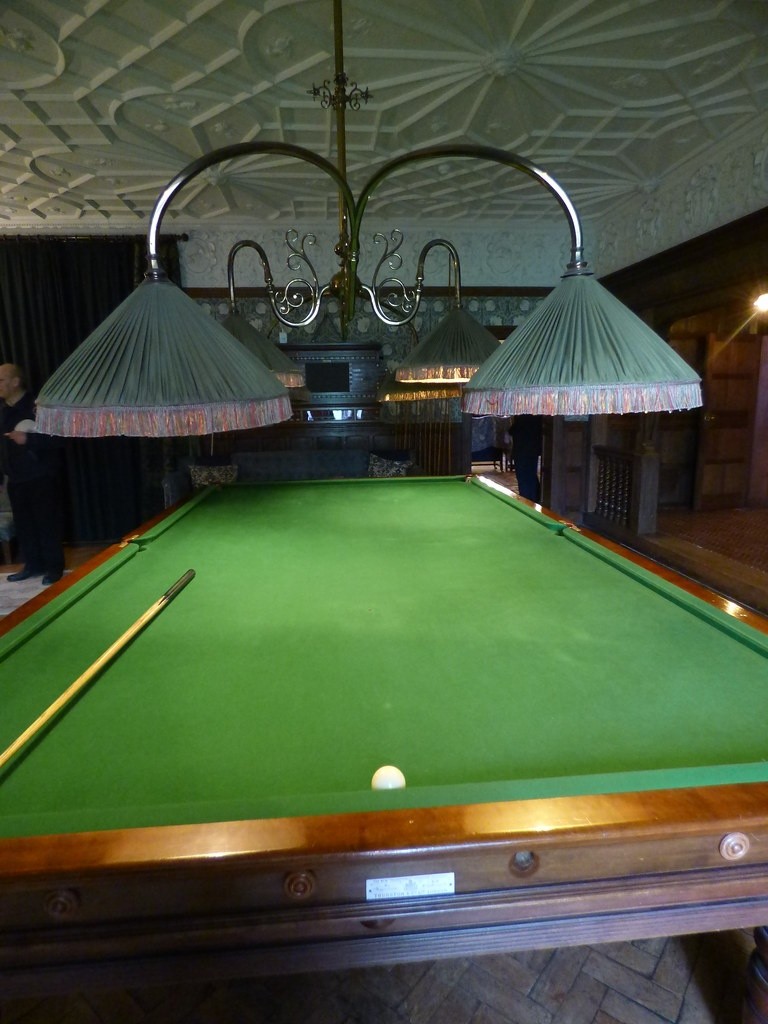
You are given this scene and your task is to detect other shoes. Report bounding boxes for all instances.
[43,572,63,584]
[7,565,41,581]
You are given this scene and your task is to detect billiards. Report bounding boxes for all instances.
[371,765,407,790]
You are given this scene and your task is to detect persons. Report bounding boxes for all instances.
[0,363,68,585]
[504,414,543,504]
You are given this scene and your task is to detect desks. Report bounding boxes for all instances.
[0,475,768,997]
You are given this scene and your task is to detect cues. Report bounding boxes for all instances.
[0,568,197,771]
[394,398,454,476]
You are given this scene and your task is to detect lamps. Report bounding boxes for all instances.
[32,0,702,438]
